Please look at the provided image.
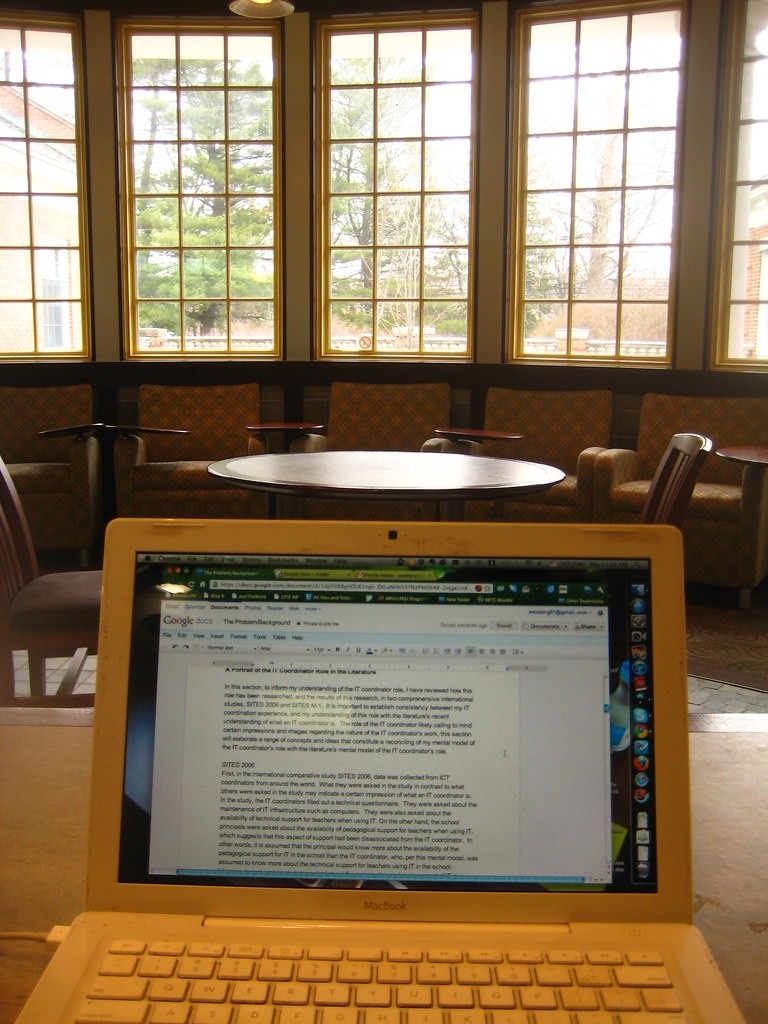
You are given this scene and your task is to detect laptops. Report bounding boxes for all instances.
[13,517,748,1024]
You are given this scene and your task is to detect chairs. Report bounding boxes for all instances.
[0,358,766,613]
[0,459,103,711]
[640,432,713,529]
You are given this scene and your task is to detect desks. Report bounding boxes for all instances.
[105,425,190,437]
[0,707,768,1024]
[433,426,524,441]
[207,451,565,521]
[245,421,324,433]
[37,423,107,435]
[714,443,766,467]
